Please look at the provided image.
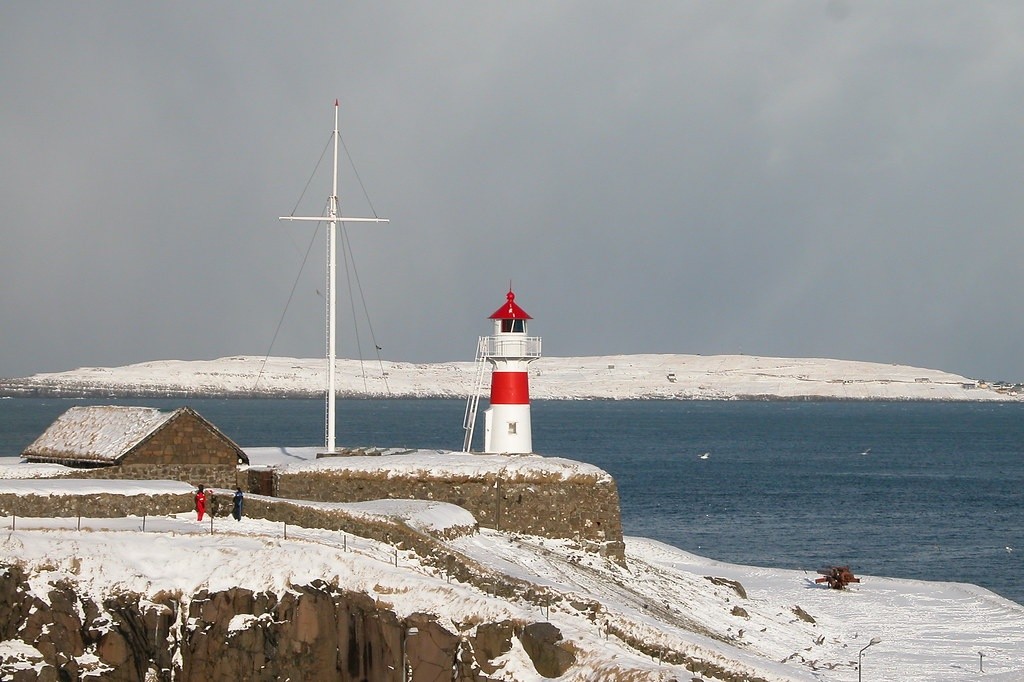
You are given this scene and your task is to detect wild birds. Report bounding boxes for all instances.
[698,452,710,459]
[860,449,871,456]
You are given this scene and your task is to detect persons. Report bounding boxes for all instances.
[233,487,243,521]
[194,484,208,521]
